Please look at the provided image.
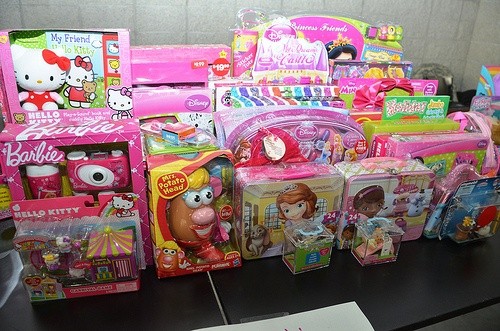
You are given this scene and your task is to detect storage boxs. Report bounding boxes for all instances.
[0,28,500,304]
